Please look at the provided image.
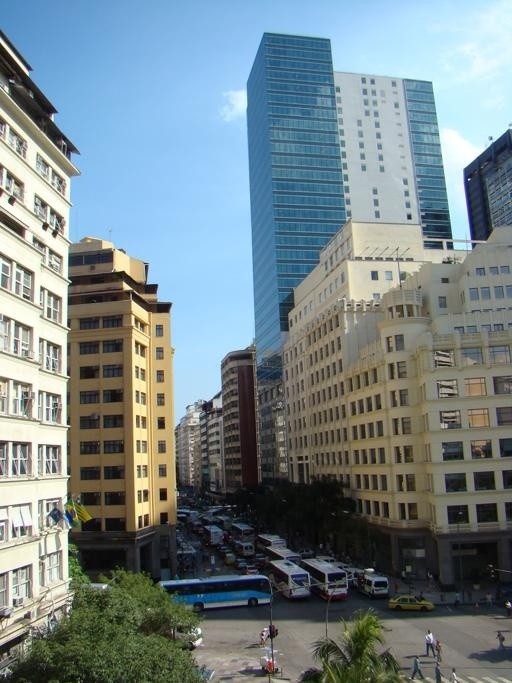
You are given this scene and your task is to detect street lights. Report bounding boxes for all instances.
[455,509,466,606]
[325,566,376,664]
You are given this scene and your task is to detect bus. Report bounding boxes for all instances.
[155,501,392,615]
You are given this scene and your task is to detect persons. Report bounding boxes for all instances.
[260,627,270,647]
[495,631,506,651]
[435,640,442,661]
[454,590,460,606]
[410,655,425,679]
[434,662,445,683]
[425,629,436,657]
[504,599,512,615]
[484,591,495,609]
[448,667,460,683]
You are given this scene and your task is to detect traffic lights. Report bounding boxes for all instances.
[269,624,279,638]
[487,561,497,579]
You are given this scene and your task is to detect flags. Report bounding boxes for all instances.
[49,497,73,529]
[64,497,82,529]
[74,497,96,523]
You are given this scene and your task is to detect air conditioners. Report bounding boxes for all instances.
[25,608,37,620]
[13,594,24,606]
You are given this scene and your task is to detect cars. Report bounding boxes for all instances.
[388,592,435,612]
[171,622,202,650]
